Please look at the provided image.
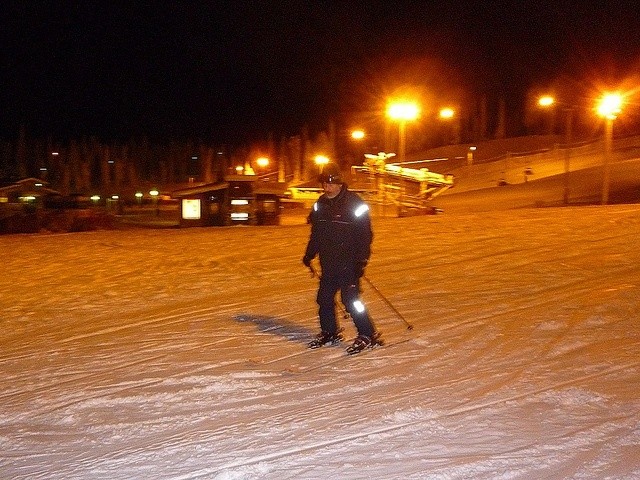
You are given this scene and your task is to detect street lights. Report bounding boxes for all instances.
[593,93,620,207]
[385,99,420,216]
[538,96,580,207]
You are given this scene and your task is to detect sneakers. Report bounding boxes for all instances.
[352,329,379,352]
[315,327,340,344]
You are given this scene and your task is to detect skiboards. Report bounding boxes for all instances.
[247,333,422,373]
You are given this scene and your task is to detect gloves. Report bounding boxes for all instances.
[355,261,367,277]
[302,255,312,268]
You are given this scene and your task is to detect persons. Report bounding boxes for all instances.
[302,162,377,355]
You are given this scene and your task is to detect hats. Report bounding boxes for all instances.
[321,163,342,183]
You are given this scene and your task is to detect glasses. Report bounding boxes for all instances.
[318,174,335,183]
